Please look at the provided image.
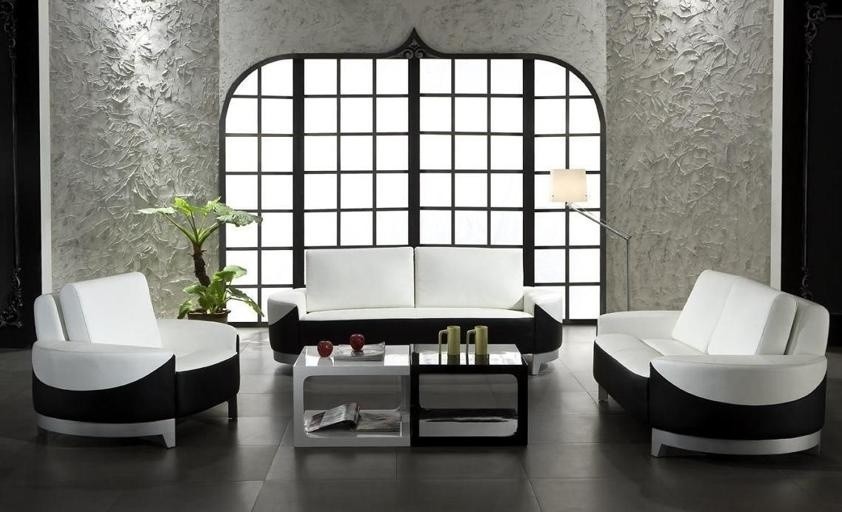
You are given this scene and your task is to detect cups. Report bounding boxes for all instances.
[438,325,461,356]
[466,325,488,356]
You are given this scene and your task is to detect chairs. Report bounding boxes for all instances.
[30,271,241,449]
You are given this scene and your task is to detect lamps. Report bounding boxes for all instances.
[547,168,634,310]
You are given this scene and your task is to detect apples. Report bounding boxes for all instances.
[318,341,332,357]
[350,334,364,350]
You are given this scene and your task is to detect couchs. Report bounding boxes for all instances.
[592,272,832,461]
[266,244,564,376]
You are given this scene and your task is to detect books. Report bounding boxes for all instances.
[334,341,387,362]
[305,401,402,434]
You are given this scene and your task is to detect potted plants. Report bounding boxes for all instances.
[129,193,268,325]
